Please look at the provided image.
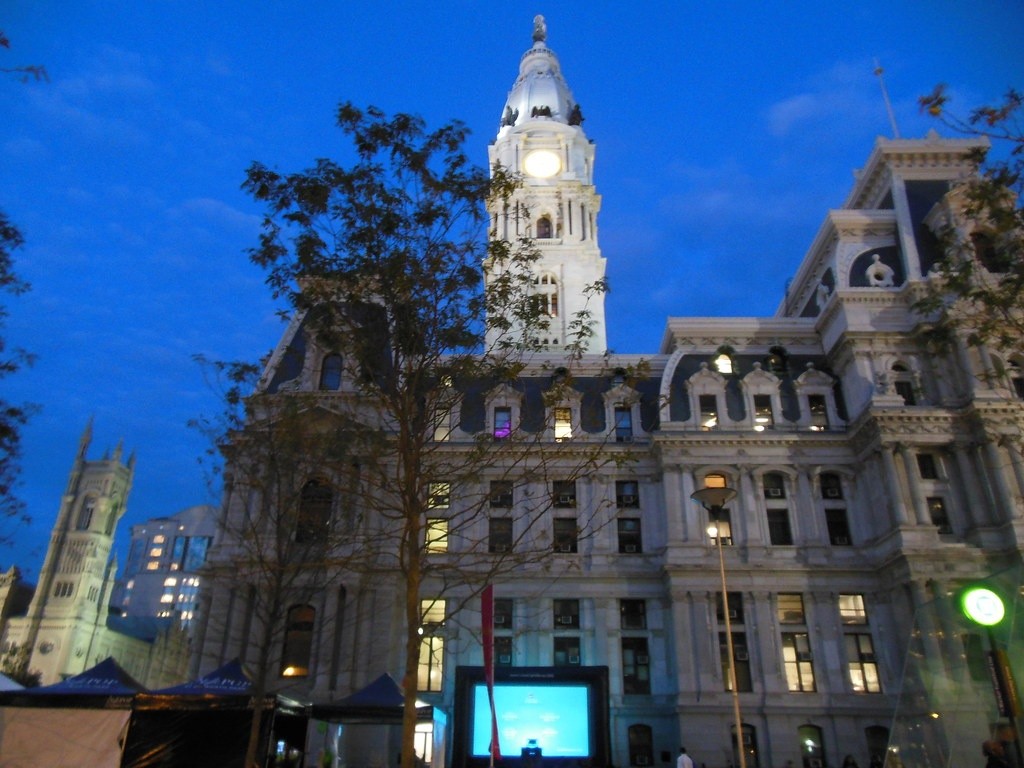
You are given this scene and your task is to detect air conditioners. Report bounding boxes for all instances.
[623,496,633,503]
[863,653,877,662]
[562,616,571,623]
[636,755,648,765]
[434,497,442,504]
[729,609,736,618]
[494,543,507,552]
[769,488,781,496]
[835,536,848,545]
[625,544,637,553]
[741,735,751,745]
[715,538,729,545]
[637,655,648,664]
[623,436,633,441]
[490,495,500,503]
[499,655,510,663]
[807,759,822,768]
[569,656,579,663]
[494,616,504,623]
[799,652,813,660]
[736,652,748,660]
[559,543,572,552]
[827,489,839,497]
[560,495,569,503]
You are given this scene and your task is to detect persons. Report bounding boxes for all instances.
[676,746,694,768]
[886,750,905,768]
[842,753,859,768]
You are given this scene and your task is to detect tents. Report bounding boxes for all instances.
[0,654,307,768]
[309,672,448,767]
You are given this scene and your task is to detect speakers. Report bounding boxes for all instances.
[662,751,671,762]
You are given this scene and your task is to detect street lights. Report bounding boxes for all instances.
[688,487,747,768]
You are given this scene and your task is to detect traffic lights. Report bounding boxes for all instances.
[947,578,1019,637]
